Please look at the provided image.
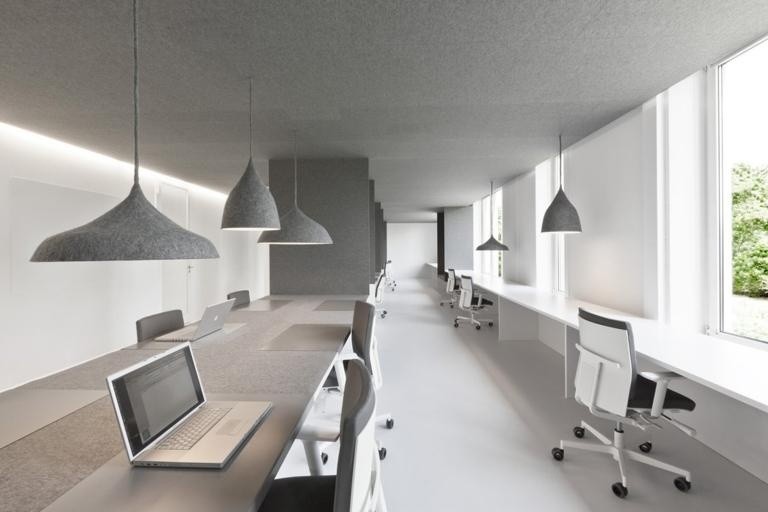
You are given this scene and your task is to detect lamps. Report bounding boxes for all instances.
[541,135,582,232]
[257,132,333,245]
[221,78,280,230]
[30,0,219,261]
[476,181,509,250]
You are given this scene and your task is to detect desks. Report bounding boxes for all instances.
[445,267,768,486]
[0,294,369,512]
[427,263,437,288]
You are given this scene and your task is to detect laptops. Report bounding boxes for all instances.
[106,340,272,469]
[153,297,236,341]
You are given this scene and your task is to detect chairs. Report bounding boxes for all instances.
[440,269,478,308]
[385,259,396,290]
[453,275,494,329]
[550,307,696,498]
[295,300,393,477]
[227,290,250,309]
[260,360,387,512]
[136,309,185,342]
[372,269,387,318]
